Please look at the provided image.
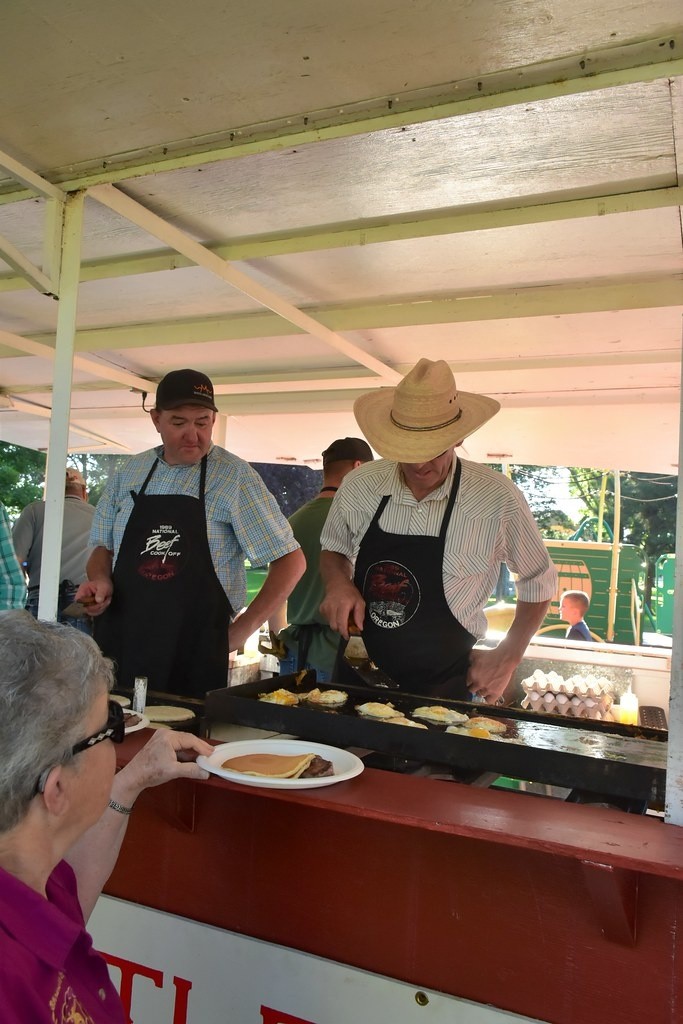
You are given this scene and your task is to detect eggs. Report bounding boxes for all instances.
[520,668,609,706]
[258,688,507,740]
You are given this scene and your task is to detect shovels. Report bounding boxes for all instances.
[344,614,400,689]
[59,596,96,618]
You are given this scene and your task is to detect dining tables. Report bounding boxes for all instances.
[98,725,681,1023]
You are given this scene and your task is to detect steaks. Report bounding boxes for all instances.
[300,755,334,781]
[121,712,141,728]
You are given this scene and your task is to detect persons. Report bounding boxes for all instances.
[73,369,306,699]
[559,591,592,652]
[319,376,555,703]
[0,608,214,1024]
[11,467,99,638]
[267,436,374,685]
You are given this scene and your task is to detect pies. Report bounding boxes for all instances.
[107,694,196,721]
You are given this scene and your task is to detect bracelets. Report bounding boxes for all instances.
[107,799,134,815]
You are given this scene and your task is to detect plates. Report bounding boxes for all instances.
[121,708,148,735]
[194,738,364,789]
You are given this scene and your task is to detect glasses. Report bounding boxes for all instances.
[36,700,125,794]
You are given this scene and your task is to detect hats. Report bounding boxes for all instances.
[65,468,86,486]
[322,438,374,465]
[154,368,219,414]
[352,357,500,465]
